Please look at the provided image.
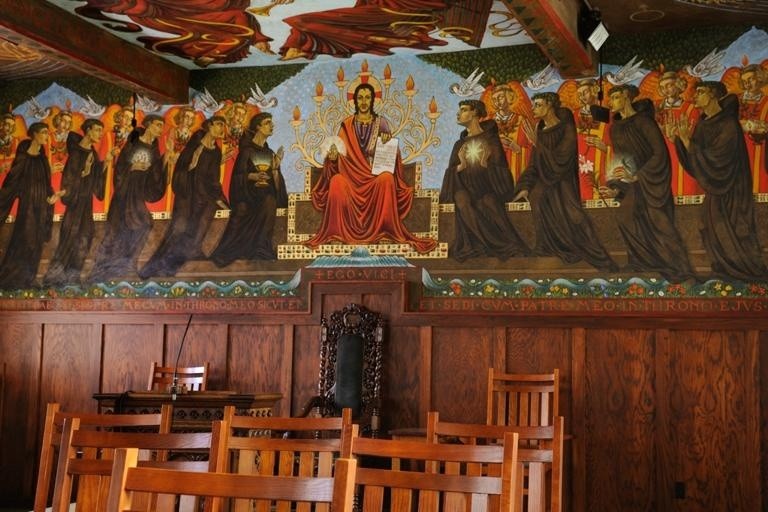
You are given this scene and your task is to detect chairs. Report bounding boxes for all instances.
[276,161,449,262]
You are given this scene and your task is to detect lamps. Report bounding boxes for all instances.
[580,13,609,52]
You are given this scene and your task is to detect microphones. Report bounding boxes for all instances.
[170,314,193,394]
